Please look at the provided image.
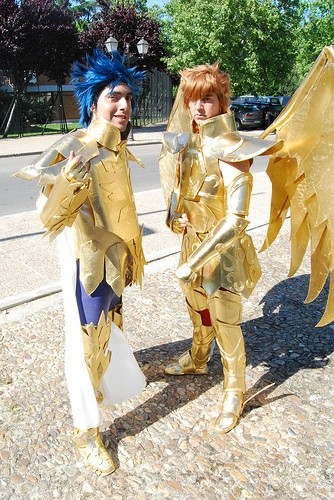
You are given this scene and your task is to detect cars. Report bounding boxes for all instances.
[229,101,264,130]
[251,102,279,128]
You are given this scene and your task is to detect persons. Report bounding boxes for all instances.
[9,47,151,477]
[157,57,285,436]
[262,104,272,130]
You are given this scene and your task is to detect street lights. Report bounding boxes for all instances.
[104,34,151,140]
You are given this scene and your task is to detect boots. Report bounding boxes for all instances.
[165,285,216,376]
[207,288,247,434]
[108,294,123,333]
[77,309,116,478]
